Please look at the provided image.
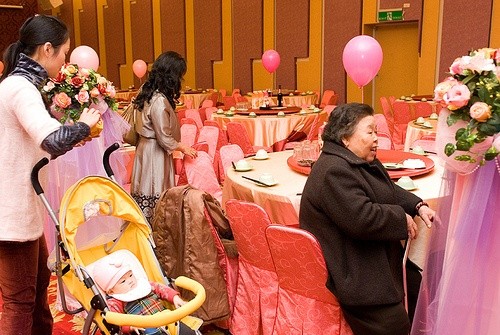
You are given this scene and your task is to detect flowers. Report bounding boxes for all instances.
[434,48,500,165]
[42,63,115,127]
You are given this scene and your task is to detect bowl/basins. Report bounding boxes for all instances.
[401,94,427,102]
[256,149,267,158]
[413,146,424,155]
[430,112,438,118]
[398,176,414,189]
[236,160,249,170]
[216,105,321,117]
[248,88,314,96]
[424,121,431,127]
[260,173,275,185]
[417,117,424,123]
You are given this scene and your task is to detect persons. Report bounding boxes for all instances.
[93,257,196,335]
[129,50,198,225]
[299,103,444,335]
[0,14,100,335]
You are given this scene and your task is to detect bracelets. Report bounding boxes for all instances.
[416,202,429,216]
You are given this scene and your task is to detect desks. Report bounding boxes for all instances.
[178,91,221,109]
[115,91,138,101]
[267,94,318,108]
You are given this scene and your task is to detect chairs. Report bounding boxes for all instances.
[174,89,439,335]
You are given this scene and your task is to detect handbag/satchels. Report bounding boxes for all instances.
[118,82,145,145]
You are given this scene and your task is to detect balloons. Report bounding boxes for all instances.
[262,50,281,73]
[70,46,99,72]
[343,35,383,87]
[133,59,147,78]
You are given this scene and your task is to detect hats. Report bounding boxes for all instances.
[93,255,132,290]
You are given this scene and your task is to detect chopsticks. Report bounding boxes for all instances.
[242,176,270,187]
[231,161,237,171]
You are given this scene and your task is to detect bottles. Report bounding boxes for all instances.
[277,85,283,107]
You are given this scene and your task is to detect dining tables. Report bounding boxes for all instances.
[213,111,321,151]
[404,119,437,158]
[394,98,438,149]
[221,150,446,271]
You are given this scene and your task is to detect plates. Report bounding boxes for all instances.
[253,157,268,160]
[422,126,433,129]
[430,118,438,120]
[252,180,277,186]
[231,166,254,171]
[414,122,424,124]
[383,162,402,169]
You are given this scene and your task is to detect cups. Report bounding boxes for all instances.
[292,139,321,166]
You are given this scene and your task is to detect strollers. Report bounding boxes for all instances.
[30,142,207,335]
